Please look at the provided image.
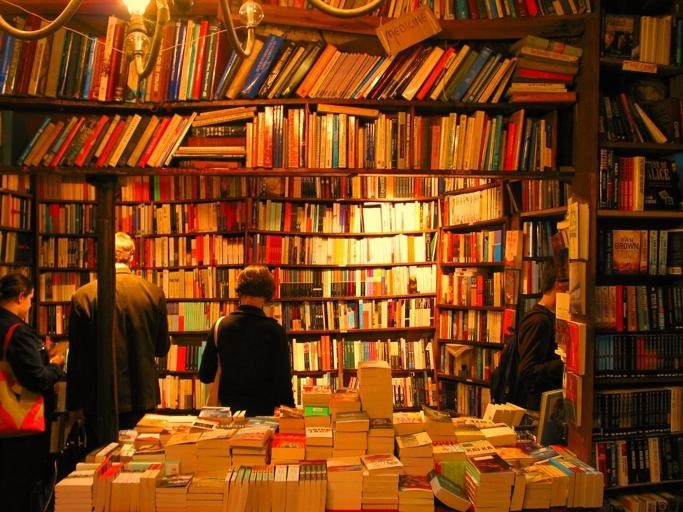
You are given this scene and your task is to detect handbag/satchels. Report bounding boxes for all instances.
[56,418,90,484]
[0,322,47,437]
[205,364,221,408]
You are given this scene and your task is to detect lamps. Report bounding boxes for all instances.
[0,1,384,77]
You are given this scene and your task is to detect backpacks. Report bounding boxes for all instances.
[490,335,521,406]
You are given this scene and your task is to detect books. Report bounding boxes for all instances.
[0,0,683,509]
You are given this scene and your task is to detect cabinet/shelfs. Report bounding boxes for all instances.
[0,0,683,500]
